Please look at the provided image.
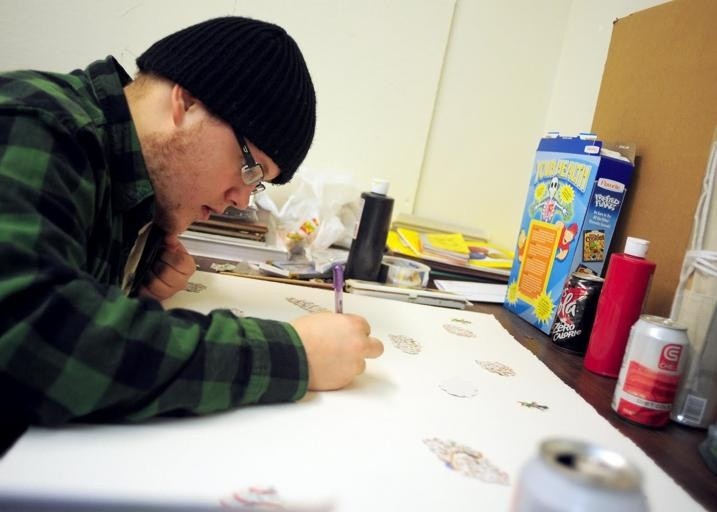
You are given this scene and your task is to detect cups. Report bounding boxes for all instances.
[382,255,432,288]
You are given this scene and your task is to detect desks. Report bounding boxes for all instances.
[1,227,717,511]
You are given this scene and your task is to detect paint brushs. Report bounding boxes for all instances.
[219,271,353,292]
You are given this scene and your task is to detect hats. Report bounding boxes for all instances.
[134,12,318,186]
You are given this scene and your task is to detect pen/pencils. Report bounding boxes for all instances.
[333,264,344,314]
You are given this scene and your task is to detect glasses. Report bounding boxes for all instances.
[222,127,267,196]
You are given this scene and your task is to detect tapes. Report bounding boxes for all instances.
[378,255,431,287]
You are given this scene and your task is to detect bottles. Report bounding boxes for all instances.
[345,178,396,280]
[583,233,657,380]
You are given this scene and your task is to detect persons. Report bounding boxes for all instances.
[0,14,384,463]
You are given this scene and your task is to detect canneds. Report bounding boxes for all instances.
[548,271,604,357]
[610,313,691,428]
